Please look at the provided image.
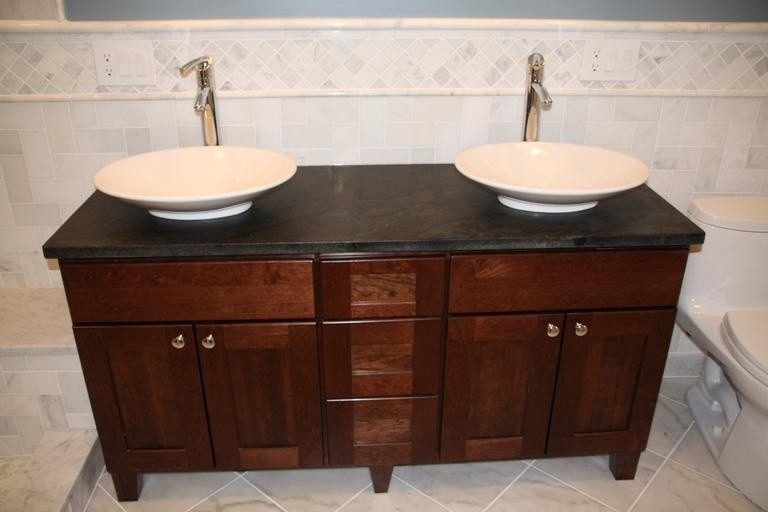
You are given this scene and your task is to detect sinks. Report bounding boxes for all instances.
[453,141,650,213]
[91,146,299,222]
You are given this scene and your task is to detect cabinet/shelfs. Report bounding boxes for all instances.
[57,258,324,474]
[317,253,448,469]
[439,247,690,465]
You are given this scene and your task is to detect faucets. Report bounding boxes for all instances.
[179,54,220,148]
[519,52,552,143]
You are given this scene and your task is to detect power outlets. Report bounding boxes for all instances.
[91,38,157,88]
[580,37,640,83]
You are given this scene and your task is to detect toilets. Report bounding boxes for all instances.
[673,194,768,512]
